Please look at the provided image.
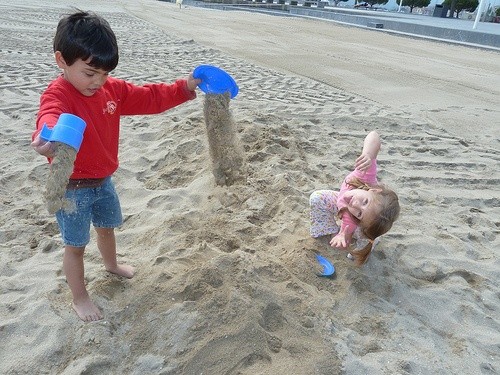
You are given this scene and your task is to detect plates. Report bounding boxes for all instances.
[316,254,334,276]
[193,64,238,99]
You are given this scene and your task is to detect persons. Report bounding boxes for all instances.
[27,6,210,320]
[308,132,402,269]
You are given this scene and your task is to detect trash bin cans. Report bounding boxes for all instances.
[432,4,445,17]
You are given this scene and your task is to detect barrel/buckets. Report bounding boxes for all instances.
[39,112,86,153]
